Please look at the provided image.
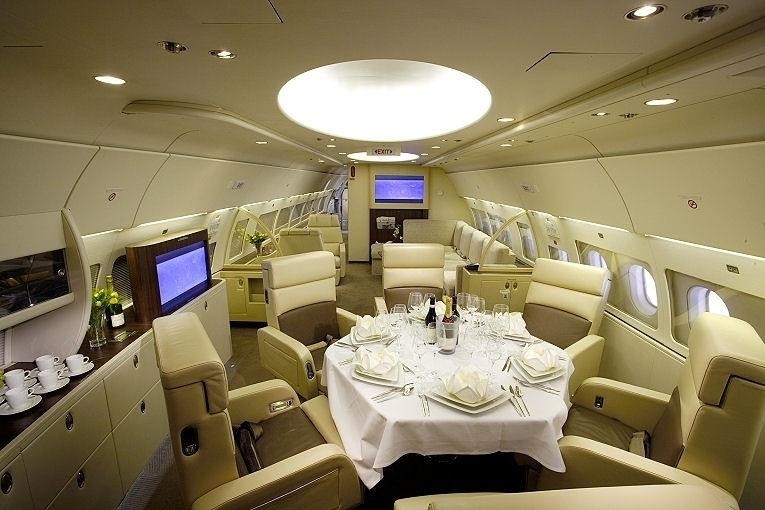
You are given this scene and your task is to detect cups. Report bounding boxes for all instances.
[3,387,34,409]
[2,368,31,388]
[36,368,63,390]
[434,313,459,357]
[64,353,89,373]
[34,354,59,371]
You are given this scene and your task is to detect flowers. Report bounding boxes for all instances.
[247,230,270,252]
[87,287,119,323]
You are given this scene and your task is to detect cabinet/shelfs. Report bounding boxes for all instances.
[0,317,166,509]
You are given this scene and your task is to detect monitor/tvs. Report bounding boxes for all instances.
[374,174,423,203]
[155,240,209,314]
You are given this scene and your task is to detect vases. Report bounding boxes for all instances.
[88,320,107,348]
[253,242,263,256]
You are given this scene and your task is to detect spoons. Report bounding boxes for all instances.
[418,385,431,418]
[369,381,416,402]
[514,386,530,416]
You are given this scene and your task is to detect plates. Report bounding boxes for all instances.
[427,382,508,415]
[0,378,37,394]
[349,353,406,389]
[504,325,534,344]
[29,378,70,395]
[513,351,567,384]
[60,361,94,378]
[348,320,391,346]
[0,394,42,415]
[29,368,40,378]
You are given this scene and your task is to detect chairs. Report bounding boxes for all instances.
[257,249,367,401]
[516,256,615,395]
[534,310,764,503]
[274,226,323,254]
[304,212,346,285]
[372,240,454,316]
[147,310,365,509]
[385,483,744,509]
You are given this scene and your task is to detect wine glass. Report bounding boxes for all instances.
[376,292,439,380]
[456,291,509,370]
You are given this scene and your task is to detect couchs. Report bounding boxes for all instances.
[403,218,513,295]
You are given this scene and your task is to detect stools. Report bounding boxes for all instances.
[371,243,384,274]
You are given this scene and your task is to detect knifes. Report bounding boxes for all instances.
[336,339,371,354]
[502,355,511,374]
[521,338,546,349]
[513,374,560,396]
[509,385,526,417]
[501,382,523,417]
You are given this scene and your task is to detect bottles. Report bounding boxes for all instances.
[441,297,458,351]
[103,275,126,330]
